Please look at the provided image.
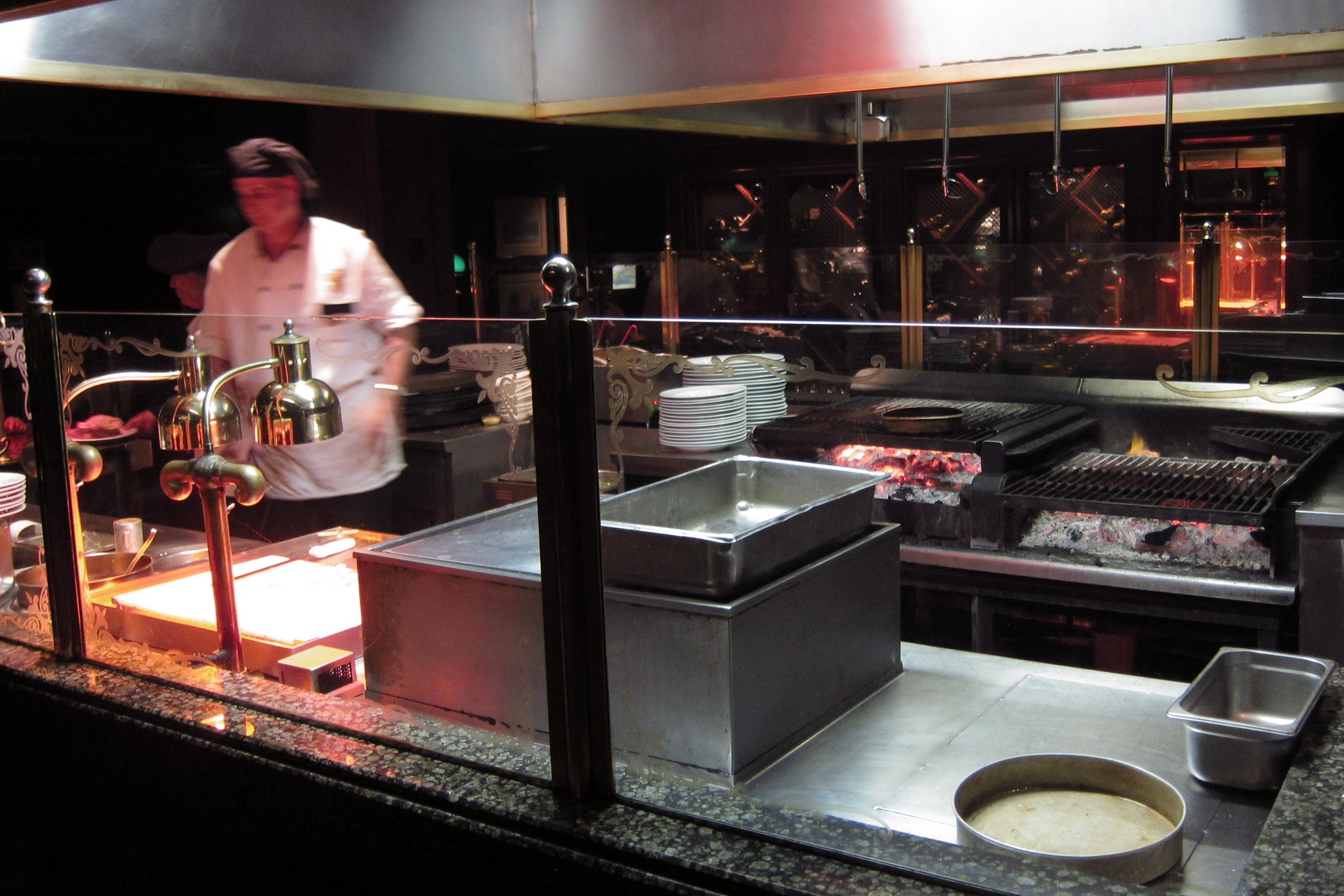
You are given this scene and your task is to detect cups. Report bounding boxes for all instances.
[113,518,143,552]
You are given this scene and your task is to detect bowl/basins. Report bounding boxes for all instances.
[482,412,500,426]
[881,407,965,433]
[951,753,1186,885]
[14,552,155,608]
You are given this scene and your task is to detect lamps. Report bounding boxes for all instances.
[160,318,344,673]
[1043,166,1056,195]
[20,336,243,493]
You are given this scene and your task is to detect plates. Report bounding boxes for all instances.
[71,426,138,445]
[682,353,788,432]
[448,343,527,370]
[658,384,747,452]
[846,328,969,369]
[494,370,533,423]
[0,472,26,518]
[1011,297,1054,312]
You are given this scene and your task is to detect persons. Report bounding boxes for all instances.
[183,139,426,544]
[124,233,229,432]
[4,415,28,434]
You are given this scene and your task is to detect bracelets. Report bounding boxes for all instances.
[374,383,410,396]
